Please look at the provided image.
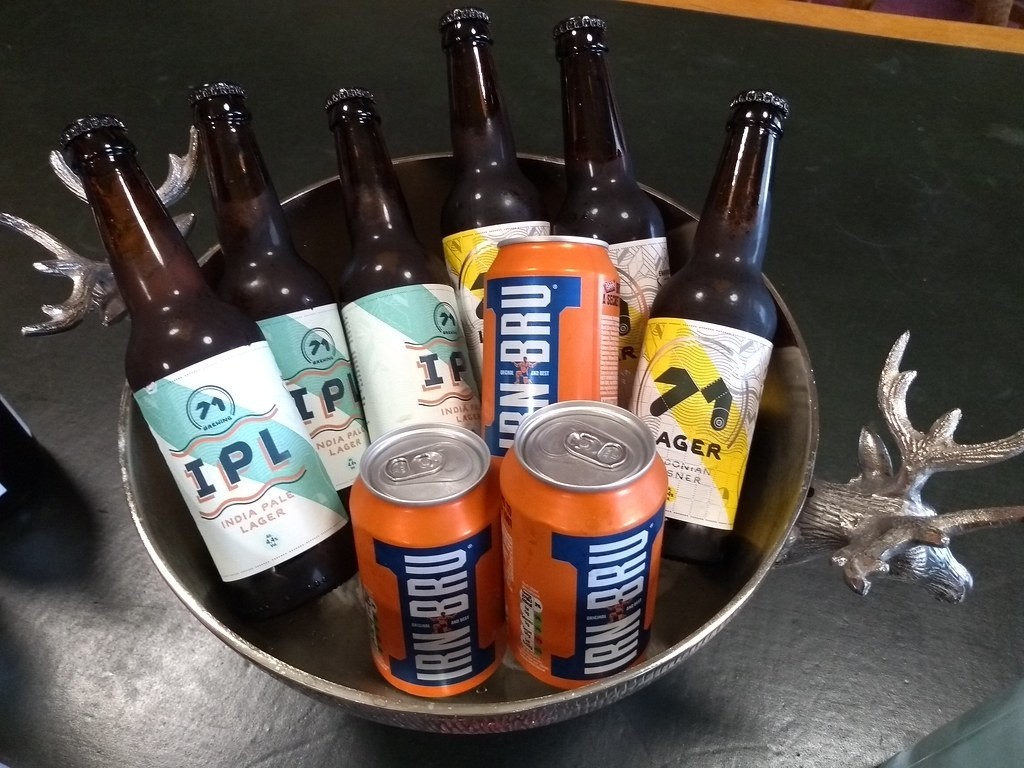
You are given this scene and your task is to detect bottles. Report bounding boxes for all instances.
[324,89,483,440]
[551,17,679,404]
[440,6,560,385]
[607,89,790,562]
[186,82,384,557]
[62,114,370,626]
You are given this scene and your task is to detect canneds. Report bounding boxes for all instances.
[499,399,667,689]
[349,425,506,697]
[481,235,621,481]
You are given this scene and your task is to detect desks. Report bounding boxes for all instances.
[0,0,1024,768]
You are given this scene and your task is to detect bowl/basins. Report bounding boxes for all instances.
[119,145,823,737]
